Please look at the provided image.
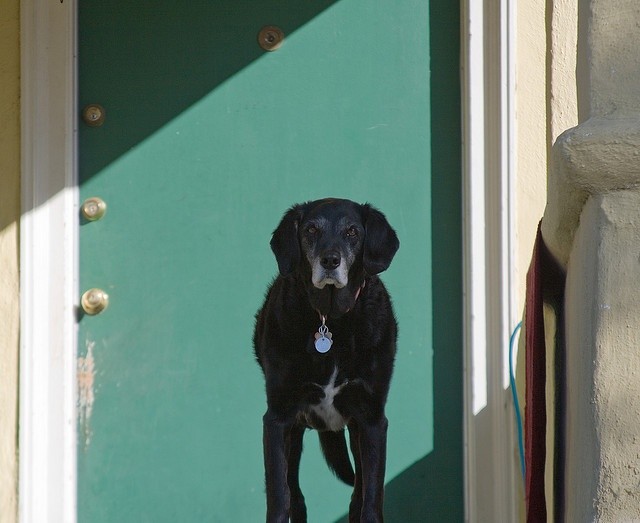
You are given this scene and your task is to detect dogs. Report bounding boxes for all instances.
[251,198,399,523]
